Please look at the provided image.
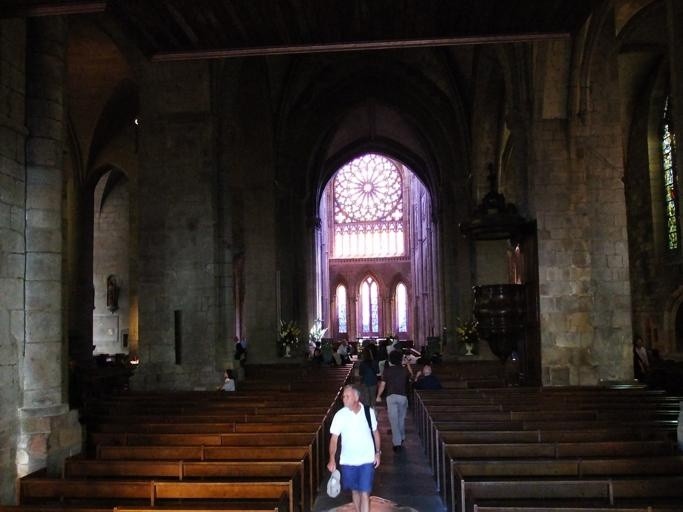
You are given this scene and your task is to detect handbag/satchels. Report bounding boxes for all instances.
[375,450,383,454]
[415,377,418,379]
[644,366,649,370]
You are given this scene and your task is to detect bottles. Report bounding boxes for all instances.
[327,469,340,498]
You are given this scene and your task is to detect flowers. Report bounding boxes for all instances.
[280,320,304,345]
[457,322,481,344]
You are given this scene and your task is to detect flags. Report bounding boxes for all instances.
[284,346,291,358]
[465,344,474,355]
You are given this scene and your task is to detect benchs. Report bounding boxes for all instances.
[406,365,683,512]
[0,364,354,512]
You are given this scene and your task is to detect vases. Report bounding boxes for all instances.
[280,320,304,345]
[284,346,291,358]
[457,322,481,344]
[465,344,474,355]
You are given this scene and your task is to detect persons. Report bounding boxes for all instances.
[358,349,381,408]
[634,336,650,373]
[240,338,246,347]
[485,331,512,377]
[219,368,236,392]
[413,364,443,390]
[336,338,349,366]
[634,351,649,382]
[506,337,520,369]
[327,383,381,511]
[375,350,414,453]
[234,336,246,367]
[313,341,321,359]
